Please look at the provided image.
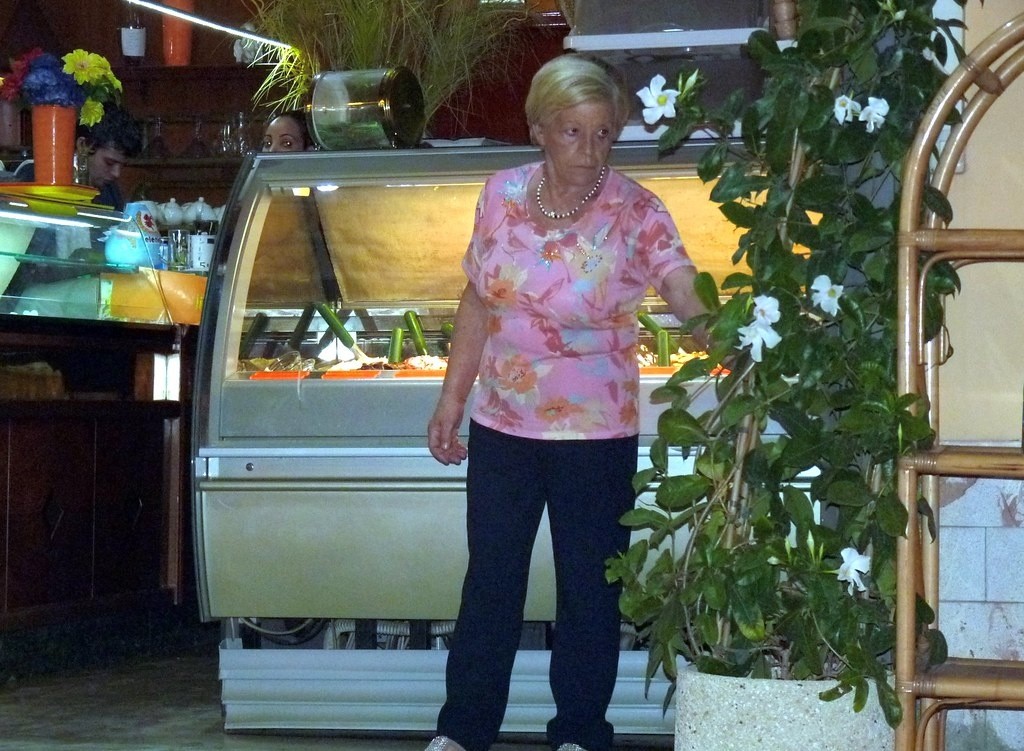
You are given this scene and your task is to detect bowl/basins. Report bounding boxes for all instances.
[266,350,301,371]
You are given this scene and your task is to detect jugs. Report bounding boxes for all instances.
[137,197,227,226]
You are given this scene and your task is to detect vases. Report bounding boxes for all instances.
[32,105,76,184]
[674,664,919,751]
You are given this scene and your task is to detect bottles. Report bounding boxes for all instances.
[115,0,147,70]
[104,203,162,270]
[140,109,251,160]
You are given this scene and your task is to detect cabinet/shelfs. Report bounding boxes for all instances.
[898,13,1024,751]
[20,63,282,233]
[0,191,189,683]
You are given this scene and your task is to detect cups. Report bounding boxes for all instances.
[168,230,192,271]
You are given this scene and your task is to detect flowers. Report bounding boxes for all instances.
[604,0,978,729]
[0,35,123,127]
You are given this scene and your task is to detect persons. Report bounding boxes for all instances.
[427,53,737,750]
[260,110,315,152]
[4,100,143,312]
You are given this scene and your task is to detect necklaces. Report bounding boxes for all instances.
[536,164,606,219]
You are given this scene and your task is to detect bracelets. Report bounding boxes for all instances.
[706,328,715,356]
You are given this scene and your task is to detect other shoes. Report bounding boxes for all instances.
[557,743,587,751]
[424,735,466,751]
